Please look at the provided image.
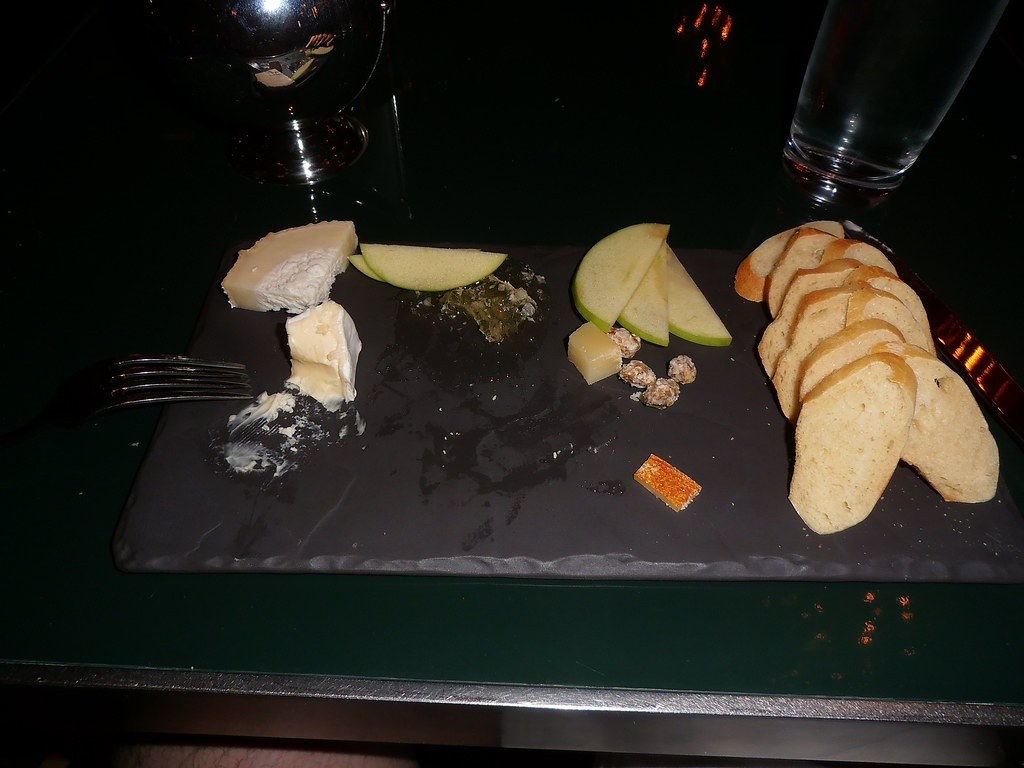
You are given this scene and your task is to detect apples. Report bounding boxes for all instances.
[348,243,507,292]
[571,223,732,347]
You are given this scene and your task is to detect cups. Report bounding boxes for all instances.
[784,0,1010,193]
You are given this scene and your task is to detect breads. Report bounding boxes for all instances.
[734,220,999,534]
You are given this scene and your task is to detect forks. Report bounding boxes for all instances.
[0,355,253,449]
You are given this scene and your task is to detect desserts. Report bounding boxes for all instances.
[285,300,362,413]
[222,220,358,315]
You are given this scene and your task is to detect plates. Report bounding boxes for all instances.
[115,241,1024,583]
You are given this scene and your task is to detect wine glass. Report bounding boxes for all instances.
[151,0,386,187]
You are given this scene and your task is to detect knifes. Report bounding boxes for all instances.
[840,219,1024,449]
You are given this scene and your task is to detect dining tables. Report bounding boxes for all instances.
[0,0,1024,729]
